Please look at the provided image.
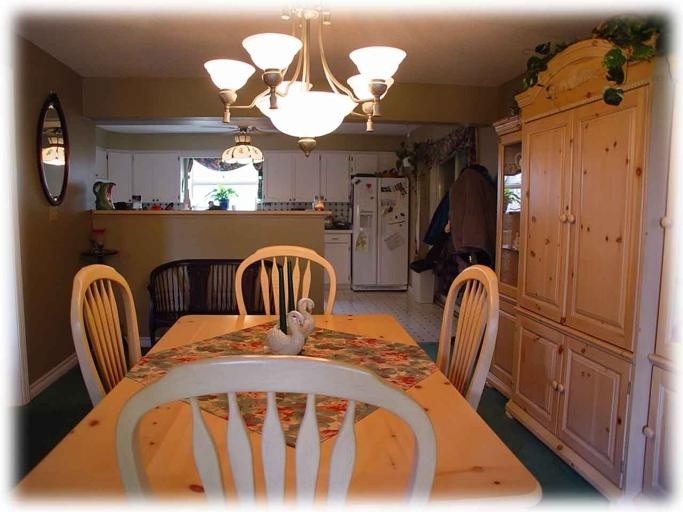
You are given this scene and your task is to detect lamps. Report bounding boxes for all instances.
[41,127,65,166]
[203,0,407,158]
[220,126,265,164]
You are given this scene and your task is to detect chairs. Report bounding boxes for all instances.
[432,263,501,413]
[113,354,438,510]
[234,244,338,315]
[69,263,142,409]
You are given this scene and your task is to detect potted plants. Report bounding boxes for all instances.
[504,178,521,214]
[204,184,239,210]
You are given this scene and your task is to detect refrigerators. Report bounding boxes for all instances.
[352,177,408,291]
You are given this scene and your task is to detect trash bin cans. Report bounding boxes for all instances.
[410,260,434,304]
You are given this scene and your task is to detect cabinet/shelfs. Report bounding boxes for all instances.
[487,116,523,400]
[514,36,659,509]
[633,51,680,509]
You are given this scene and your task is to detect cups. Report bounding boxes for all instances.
[131,195,142,210]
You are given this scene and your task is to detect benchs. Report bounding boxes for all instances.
[146,259,289,348]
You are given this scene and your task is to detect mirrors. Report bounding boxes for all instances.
[500,139,523,290]
[35,89,69,206]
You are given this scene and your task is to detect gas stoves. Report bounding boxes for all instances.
[325,220,352,230]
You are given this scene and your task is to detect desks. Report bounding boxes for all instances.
[5,315,544,510]
